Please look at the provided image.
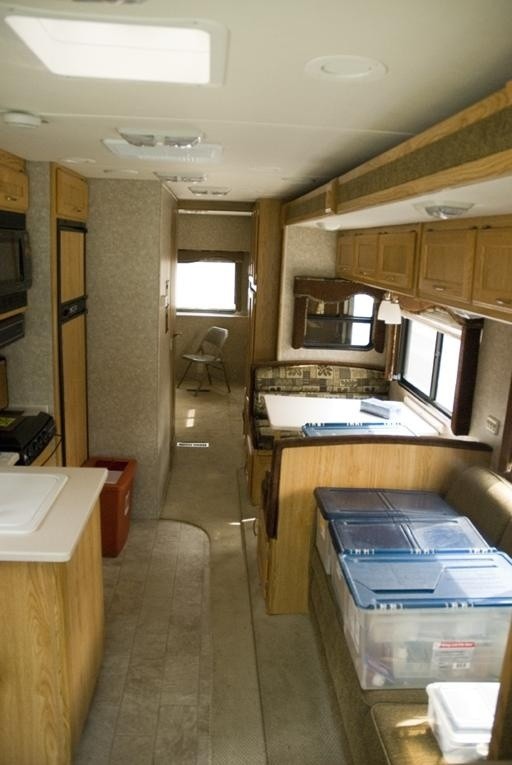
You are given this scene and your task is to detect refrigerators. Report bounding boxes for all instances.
[151,182,180,521]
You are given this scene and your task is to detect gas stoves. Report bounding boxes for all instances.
[0,408,56,466]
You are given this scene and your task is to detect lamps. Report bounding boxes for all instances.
[315,305,325,314]
[115,127,204,150]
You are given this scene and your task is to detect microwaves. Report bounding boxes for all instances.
[0,228,33,296]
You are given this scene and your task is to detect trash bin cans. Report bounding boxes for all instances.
[80,456,137,557]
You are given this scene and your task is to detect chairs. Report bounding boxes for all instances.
[177,325,232,397]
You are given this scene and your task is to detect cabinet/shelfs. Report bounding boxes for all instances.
[336,213,512,325]
[0,151,103,765]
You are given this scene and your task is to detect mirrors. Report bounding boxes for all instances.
[293,275,385,351]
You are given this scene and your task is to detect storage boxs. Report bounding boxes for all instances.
[424,677,492,757]
[328,513,491,553]
[335,549,512,694]
[317,487,459,520]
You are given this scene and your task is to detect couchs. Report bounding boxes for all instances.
[309,462,512,765]
[244,365,512,615]
